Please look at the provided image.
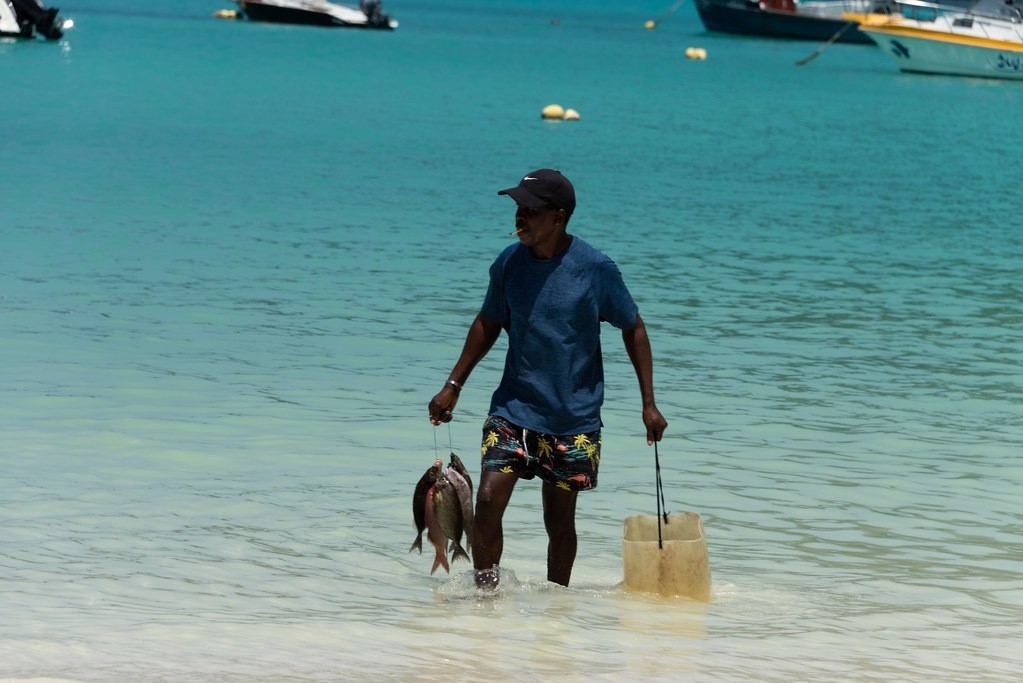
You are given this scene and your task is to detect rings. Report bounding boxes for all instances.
[429,414,433,418]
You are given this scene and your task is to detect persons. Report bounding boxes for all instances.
[428,168,668,591]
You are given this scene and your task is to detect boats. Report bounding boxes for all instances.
[228,0,399,32]
[838,0,1022,82]
[0,0,63,40]
[692,0,936,45]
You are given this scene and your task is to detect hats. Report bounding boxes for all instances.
[498,169,576,213]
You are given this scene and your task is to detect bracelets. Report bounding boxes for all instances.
[446,380,462,391]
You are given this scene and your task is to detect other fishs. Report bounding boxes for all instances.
[409,451,474,576]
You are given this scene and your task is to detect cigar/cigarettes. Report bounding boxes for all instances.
[509,229,522,236]
